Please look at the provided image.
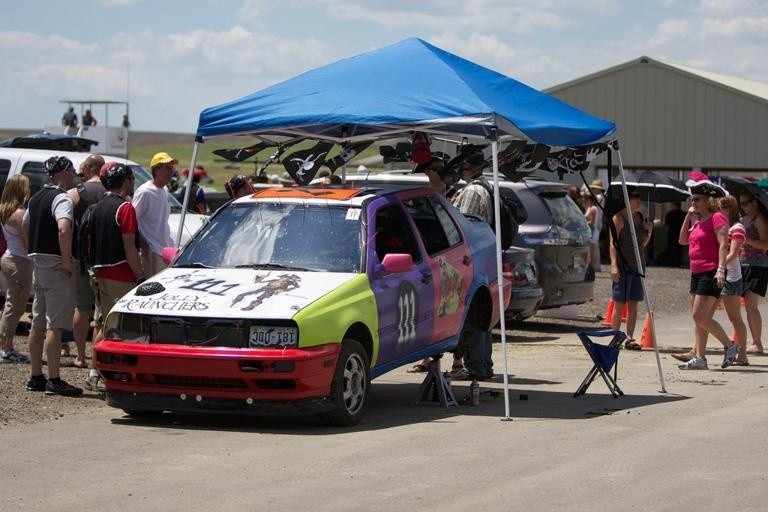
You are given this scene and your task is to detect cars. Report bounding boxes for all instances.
[91,184,511,428]
[498,244,544,330]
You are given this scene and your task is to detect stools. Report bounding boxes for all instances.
[572,329,627,399]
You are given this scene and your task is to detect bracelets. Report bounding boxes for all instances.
[717,268,725,272]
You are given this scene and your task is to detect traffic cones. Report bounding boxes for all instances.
[601,298,614,325]
[640,311,656,350]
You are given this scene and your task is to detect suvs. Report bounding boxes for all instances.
[306,170,596,326]
[0,147,211,314]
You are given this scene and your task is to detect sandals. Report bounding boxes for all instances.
[625,340,641,349]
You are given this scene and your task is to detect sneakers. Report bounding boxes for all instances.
[745,345,764,356]
[678,357,708,370]
[721,342,742,368]
[61,344,69,356]
[25,376,48,390]
[73,358,88,368]
[84,373,106,392]
[0,350,30,363]
[45,379,83,397]
[452,370,487,380]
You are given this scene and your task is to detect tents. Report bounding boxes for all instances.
[176,37,665,422]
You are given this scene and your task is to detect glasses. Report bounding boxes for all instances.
[128,177,135,180]
[691,196,705,202]
[633,194,639,198]
[428,167,438,171]
[161,165,175,168]
[740,199,752,205]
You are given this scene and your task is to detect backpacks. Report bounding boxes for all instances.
[473,175,528,251]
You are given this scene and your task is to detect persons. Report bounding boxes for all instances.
[569,191,598,271]
[671,196,748,365]
[449,149,494,381]
[83,110,97,126]
[609,187,653,351]
[132,152,179,279]
[587,179,604,236]
[423,160,457,201]
[679,172,743,370]
[21,156,83,397]
[180,167,207,215]
[663,202,686,267]
[78,164,146,391]
[61,107,78,127]
[66,155,109,378]
[0,175,34,363]
[738,192,768,354]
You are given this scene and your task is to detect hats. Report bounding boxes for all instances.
[150,152,178,169]
[182,168,207,177]
[100,162,127,188]
[590,179,604,189]
[459,150,487,168]
[44,156,71,183]
[225,173,249,198]
[685,180,729,199]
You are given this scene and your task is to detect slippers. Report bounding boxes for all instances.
[452,365,464,373]
[407,365,429,372]
[732,360,749,366]
[671,348,691,361]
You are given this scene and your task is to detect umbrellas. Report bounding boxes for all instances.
[605,172,690,221]
[717,175,768,210]
